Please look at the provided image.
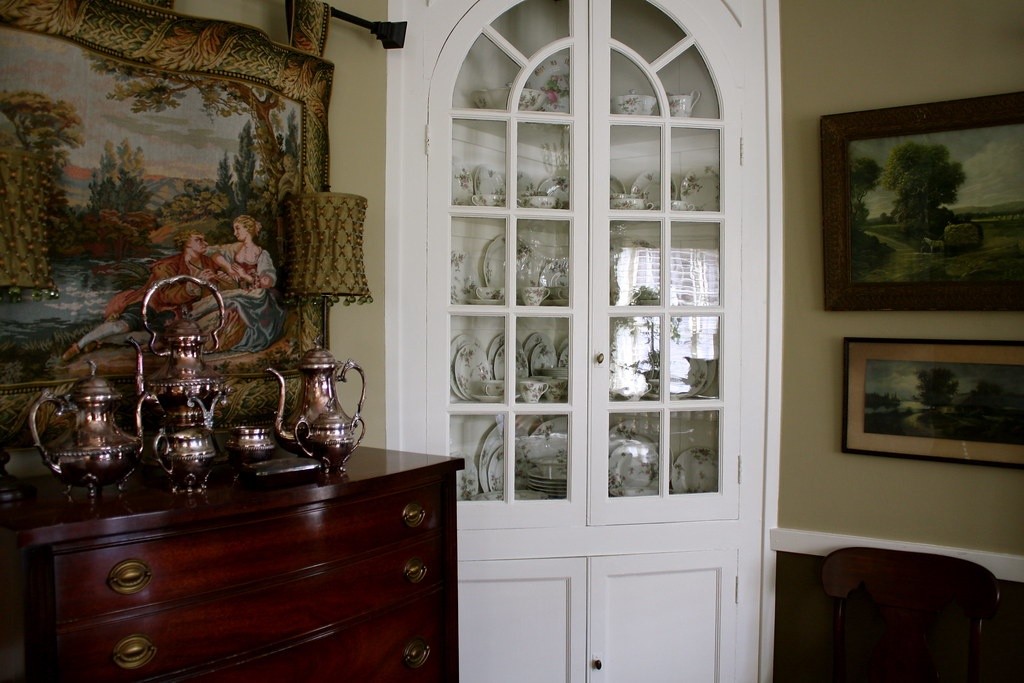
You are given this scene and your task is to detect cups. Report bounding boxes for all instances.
[544,379,568,401]
[519,381,548,403]
[518,196,558,209]
[480,380,504,396]
[470,193,506,207]
[609,196,655,211]
[226,426,274,462]
[521,286,550,306]
[668,89,700,117]
[671,199,695,212]
[473,286,504,299]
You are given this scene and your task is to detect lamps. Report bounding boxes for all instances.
[282,192,373,352]
[0,146,59,304]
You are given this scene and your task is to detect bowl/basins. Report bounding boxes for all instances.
[470,86,548,110]
[610,90,658,115]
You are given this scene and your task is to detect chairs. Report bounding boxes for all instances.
[820,546,1000,683]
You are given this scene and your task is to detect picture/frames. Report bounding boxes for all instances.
[841,336,1024,471]
[0,0,337,448]
[820,89,1024,312]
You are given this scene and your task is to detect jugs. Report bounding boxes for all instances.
[30,361,150,498]
[267,346,365,472]
[126,276,225,419]
[153,390,222,495]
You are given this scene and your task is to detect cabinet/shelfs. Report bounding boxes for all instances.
[385,0,782,683]
[0,447,464,683]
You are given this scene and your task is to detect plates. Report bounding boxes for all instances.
[524,49,569,113]
[612,237,719,305]
[609,331,718,399]
[451,233,569,306]
[449,330,569,402]
[608,421,718,497]
[452,165,570,209]
[450,413,567,500]
[610,165,719,211]
